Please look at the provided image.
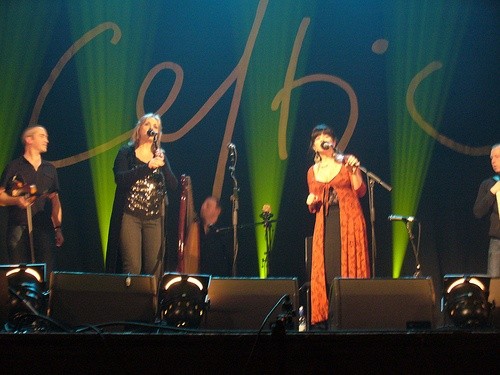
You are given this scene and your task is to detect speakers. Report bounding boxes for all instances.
[327,278,433,331]
[207,277,298,328]
[48,272,157,335]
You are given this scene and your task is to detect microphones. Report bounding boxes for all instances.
[388,215,415,223]
[147,129,156,135]
[320,142,331,149]
[227,143,236,150]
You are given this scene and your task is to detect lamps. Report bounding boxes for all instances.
[442,274,496,332]
[157,271,212,330]
[0,262,51,334]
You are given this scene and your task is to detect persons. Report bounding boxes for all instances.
[306,124,370,328]
[0,125,64,281]
[106,114,166,276]
[184,197,222,274]
[473,144,500,307]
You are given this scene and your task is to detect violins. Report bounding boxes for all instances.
[6,180,59,200]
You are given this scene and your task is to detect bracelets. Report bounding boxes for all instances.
[54,226,61,229]
